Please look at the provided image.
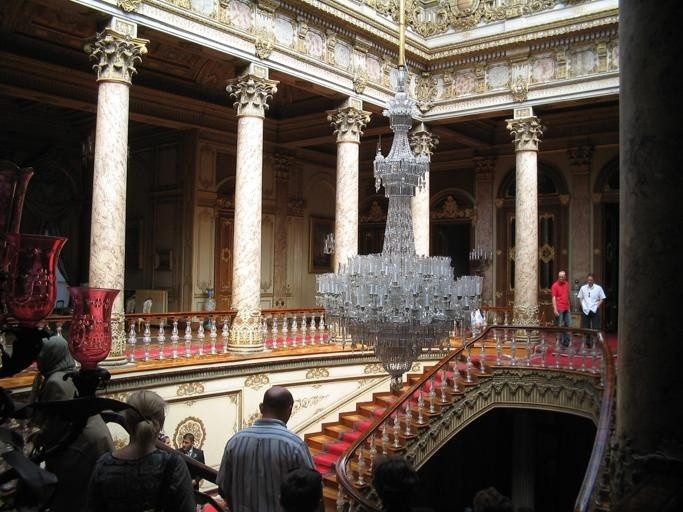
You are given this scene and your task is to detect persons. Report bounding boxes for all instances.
[215,385,315,512]
[370,455,421,511]
[158,432,172,448]
[278,466,323,512]
[86,390,196,512]
[177,433,205,493]
[29,333,114,511]
[576,273,606,356]
[471,485,510,512]
[550,271,572,352]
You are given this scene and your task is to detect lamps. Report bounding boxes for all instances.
[313,1,486,393]
[0,155,121,511]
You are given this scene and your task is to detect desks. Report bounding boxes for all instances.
[570,312,585,336]
